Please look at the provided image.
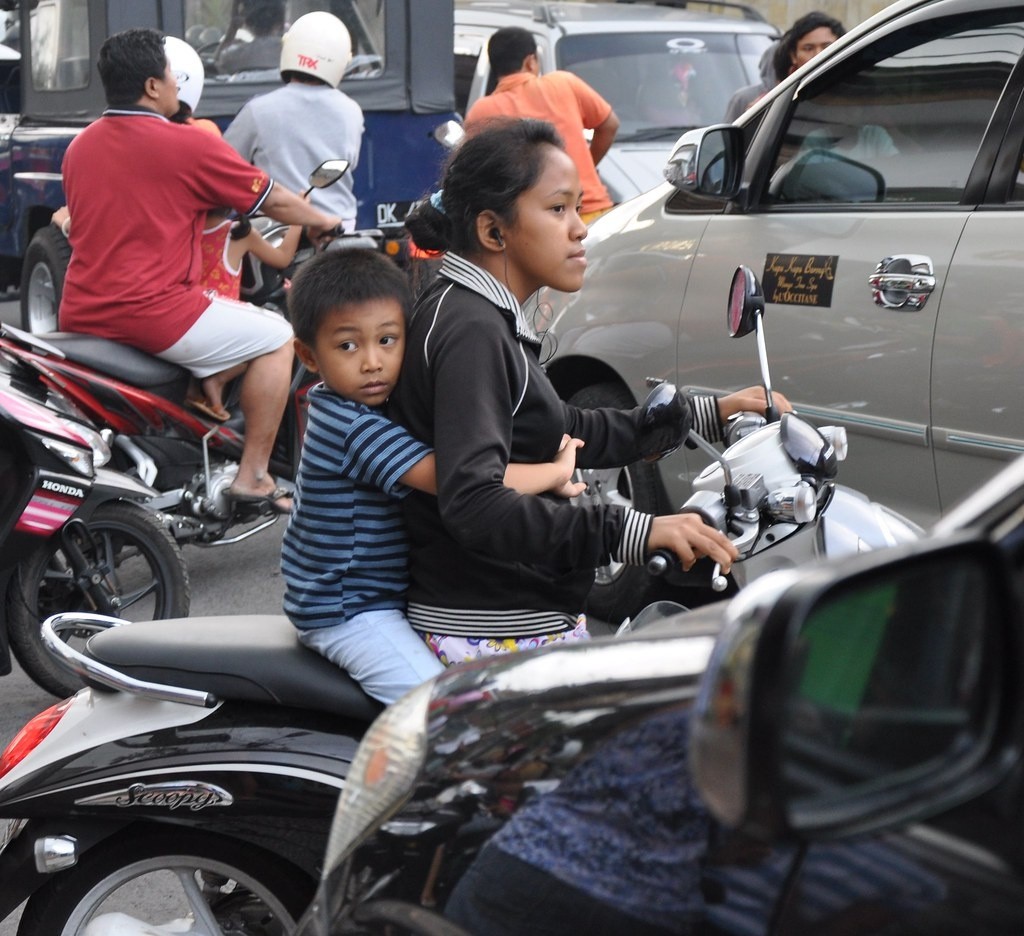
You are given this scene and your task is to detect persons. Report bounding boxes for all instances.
[58,27,344,511]
[52,35,225,236]
[799,101,901,200]
[726,11,848,121]
[290,253,590,888]
[188,189,313,421]
[221,9,367,240]
[388,116,795,660]
[459,26,621,224]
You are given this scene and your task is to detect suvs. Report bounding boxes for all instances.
[451,1,1024,520]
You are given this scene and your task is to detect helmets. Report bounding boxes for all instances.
[280,11,351,89]
[162,36,204,114]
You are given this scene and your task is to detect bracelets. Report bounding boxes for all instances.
[62,215,73,239]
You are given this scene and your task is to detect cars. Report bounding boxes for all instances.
[312,456,1024,932]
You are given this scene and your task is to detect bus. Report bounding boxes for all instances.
[0,0,465,359]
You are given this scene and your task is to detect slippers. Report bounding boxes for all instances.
[221,486,294,513]
[187,396,231,420]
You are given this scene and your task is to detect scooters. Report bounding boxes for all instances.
[0,265,931,936]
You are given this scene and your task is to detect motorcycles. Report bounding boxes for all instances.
[0,120,461,701]
